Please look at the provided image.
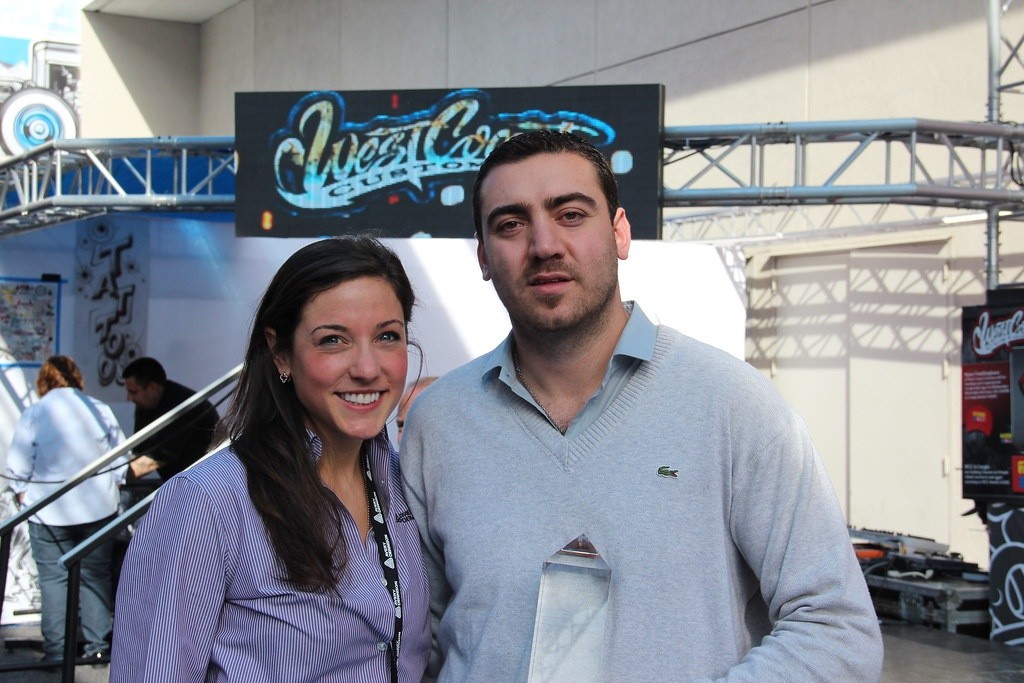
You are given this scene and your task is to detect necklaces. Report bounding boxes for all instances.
[511,306,634,436]
[357,446,372,538]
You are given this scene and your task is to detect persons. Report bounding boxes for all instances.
[395,123,889,681]
[6,356,135,670]
[107,234,431,681]
[117,356,229,484]
